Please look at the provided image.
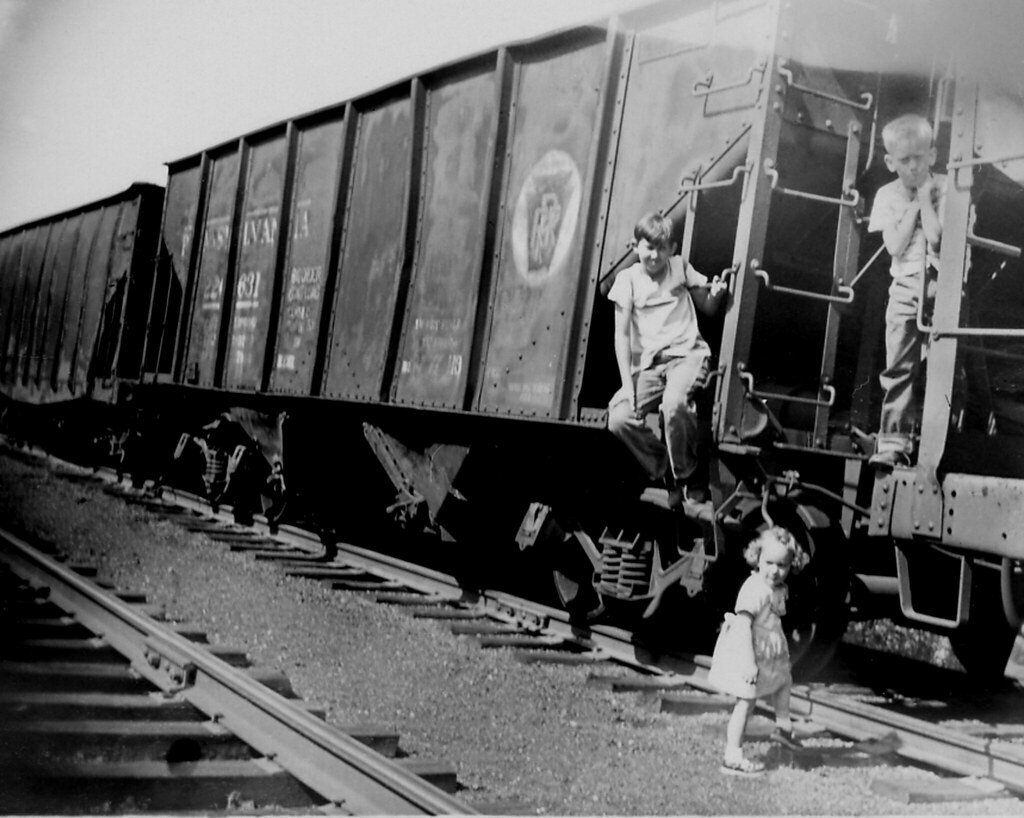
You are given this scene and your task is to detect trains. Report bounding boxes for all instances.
[0,1,1024,720]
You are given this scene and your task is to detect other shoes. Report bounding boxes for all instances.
[720,759,767,777]
[868,451,901,467]
[687,484,706,502]
[668,487,682,506]
[770,727,805,752]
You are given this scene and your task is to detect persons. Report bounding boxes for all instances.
[708,527,803,772]
[608,213,728,505]
[866,113,945,467]
